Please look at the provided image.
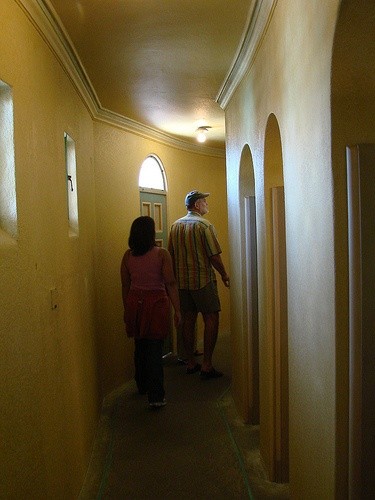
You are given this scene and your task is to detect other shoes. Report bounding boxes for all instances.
[199,362,223,380]
[178,359,197,369]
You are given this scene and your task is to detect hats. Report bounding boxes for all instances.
[185,190,210,206]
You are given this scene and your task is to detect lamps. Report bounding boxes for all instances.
[196,127,208,143]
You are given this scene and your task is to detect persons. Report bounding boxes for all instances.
[121,217,180,407]
[167,192,231,380]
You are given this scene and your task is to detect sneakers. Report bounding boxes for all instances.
[148,397,168,408]
